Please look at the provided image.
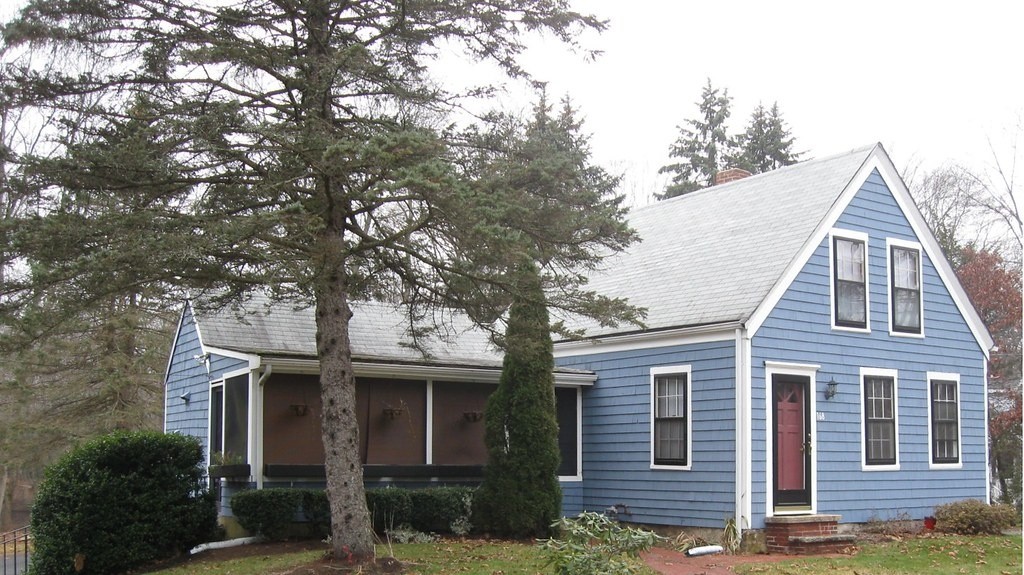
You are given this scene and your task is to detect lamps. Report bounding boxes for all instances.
[193,354,208,364]
[825,375,839,399]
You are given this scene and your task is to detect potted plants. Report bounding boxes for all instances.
[383,398,415,439]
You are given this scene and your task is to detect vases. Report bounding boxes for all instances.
[464,411,483,422]
[290,404,313,415]
